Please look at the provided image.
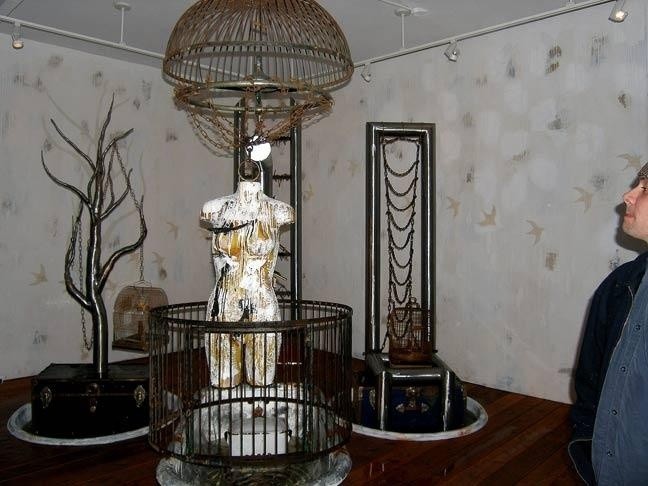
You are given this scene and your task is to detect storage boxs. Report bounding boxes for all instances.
[27,359,153,436]
[352,351,468,434]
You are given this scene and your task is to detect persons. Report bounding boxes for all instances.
[199,182,296,388]
[567,164,648,486]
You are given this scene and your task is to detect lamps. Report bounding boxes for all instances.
[356,0,645,82]
[7,17,28,48]
